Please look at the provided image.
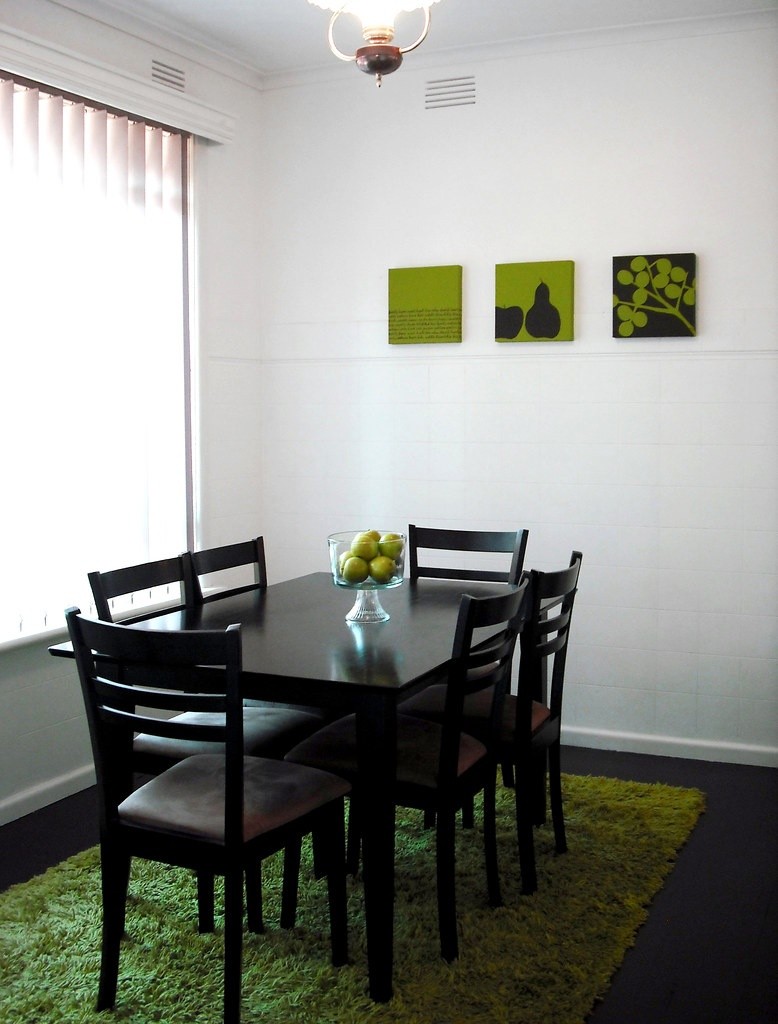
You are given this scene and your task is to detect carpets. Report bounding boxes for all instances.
[0,769,714,1024]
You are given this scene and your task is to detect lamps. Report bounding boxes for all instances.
[309,0,439,89]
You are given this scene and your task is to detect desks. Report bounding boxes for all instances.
[49,570,524,1003]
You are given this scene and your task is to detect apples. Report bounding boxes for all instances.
[339,528,402,582]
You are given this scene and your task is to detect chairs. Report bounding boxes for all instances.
[175,536,349,724]
[63,607,347,1023]
[407,523,529,826]
[277,578,528,965]
[395,550,585,891]
[85,550,320,932]
